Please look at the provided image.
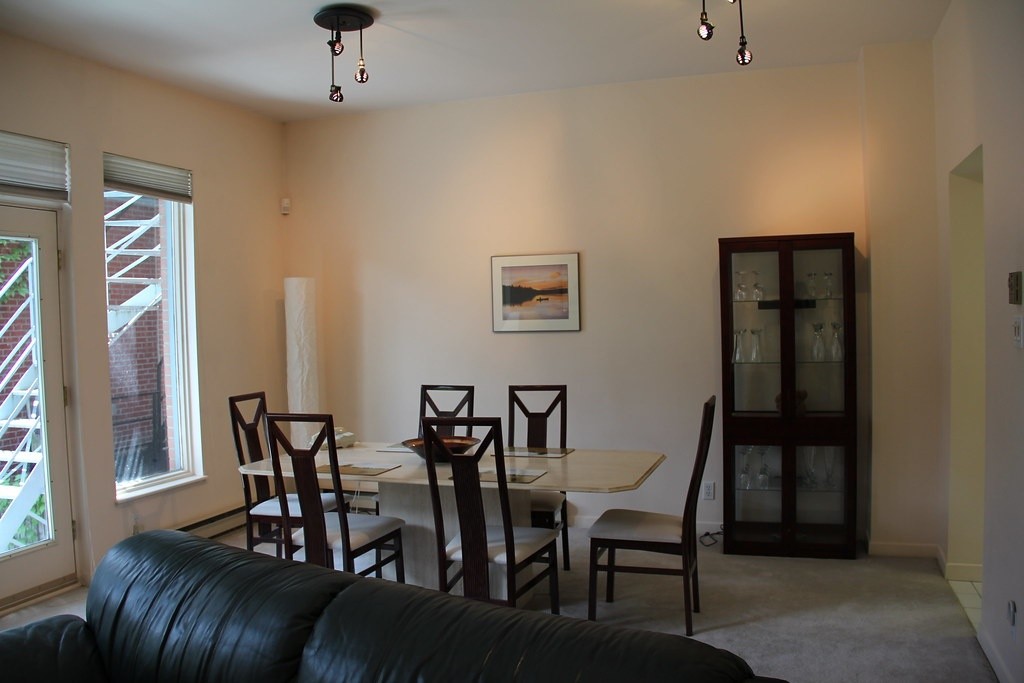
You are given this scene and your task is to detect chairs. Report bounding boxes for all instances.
[228,391,351,561]
[507,385,571,572]
[373,384,474,517]
[421,416,560,616]
[586,395,717,637]
[263,412,407,584]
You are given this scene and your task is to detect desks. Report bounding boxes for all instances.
[237,441,667,611]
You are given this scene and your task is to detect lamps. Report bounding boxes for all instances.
[696,0,752,66]
[312,6,374,101]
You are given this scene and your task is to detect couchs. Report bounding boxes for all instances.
[0,528,790,682]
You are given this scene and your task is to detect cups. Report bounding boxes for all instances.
[733,328,748,363]
[750,328,763,363]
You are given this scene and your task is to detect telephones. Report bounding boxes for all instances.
[310,426,356,451]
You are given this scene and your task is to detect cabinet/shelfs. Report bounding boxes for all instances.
[717,230,872,560]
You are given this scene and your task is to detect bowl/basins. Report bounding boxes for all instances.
[402,436,481,463]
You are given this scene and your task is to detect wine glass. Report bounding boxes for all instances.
[755,446,772,490]
[806,272,818,299]
[735,270,747,301]
[823,446,841,489]
[802,446,819,488]
[822,272,837,298]
[750,270,766,301]
[736,445,754,491]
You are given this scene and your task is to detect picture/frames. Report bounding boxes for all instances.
[490,253,580,333]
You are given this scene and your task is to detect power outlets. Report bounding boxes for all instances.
[702,480,716,501]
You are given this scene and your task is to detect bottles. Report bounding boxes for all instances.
[810,321,826,361]
[830,320,845,361]
